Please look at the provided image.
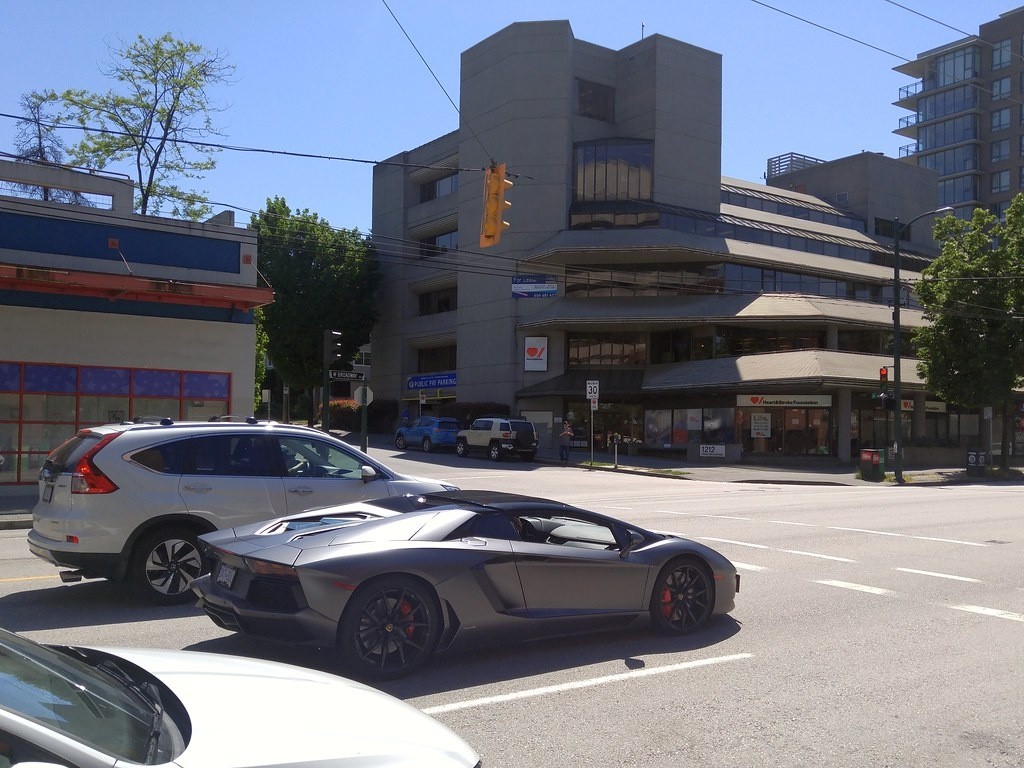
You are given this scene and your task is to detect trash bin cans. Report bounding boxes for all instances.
[968,446,986,478]
[860,449,885,483]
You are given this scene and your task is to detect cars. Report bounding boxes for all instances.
[1,627,484,768]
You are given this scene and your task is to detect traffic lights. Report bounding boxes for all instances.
[879,367,888,392]
[327,329,342,361]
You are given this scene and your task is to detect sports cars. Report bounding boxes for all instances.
[188,490,741,684]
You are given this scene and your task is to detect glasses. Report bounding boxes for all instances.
[565,426,569,427]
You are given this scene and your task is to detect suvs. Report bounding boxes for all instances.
[456,417,539,462]
[28,416,462,606]
[394,415,464,454]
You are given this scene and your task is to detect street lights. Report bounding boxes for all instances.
[893,206,956,484]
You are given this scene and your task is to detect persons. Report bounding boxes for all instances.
[255,439,307,474]
[472,514,524,541]
[463,414,473,430]
[558,421,573,463]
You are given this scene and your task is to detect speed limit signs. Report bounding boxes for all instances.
[587,380,599,400]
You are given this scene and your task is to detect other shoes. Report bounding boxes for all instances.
[561,461,564,464]
[565,461,568,463]
